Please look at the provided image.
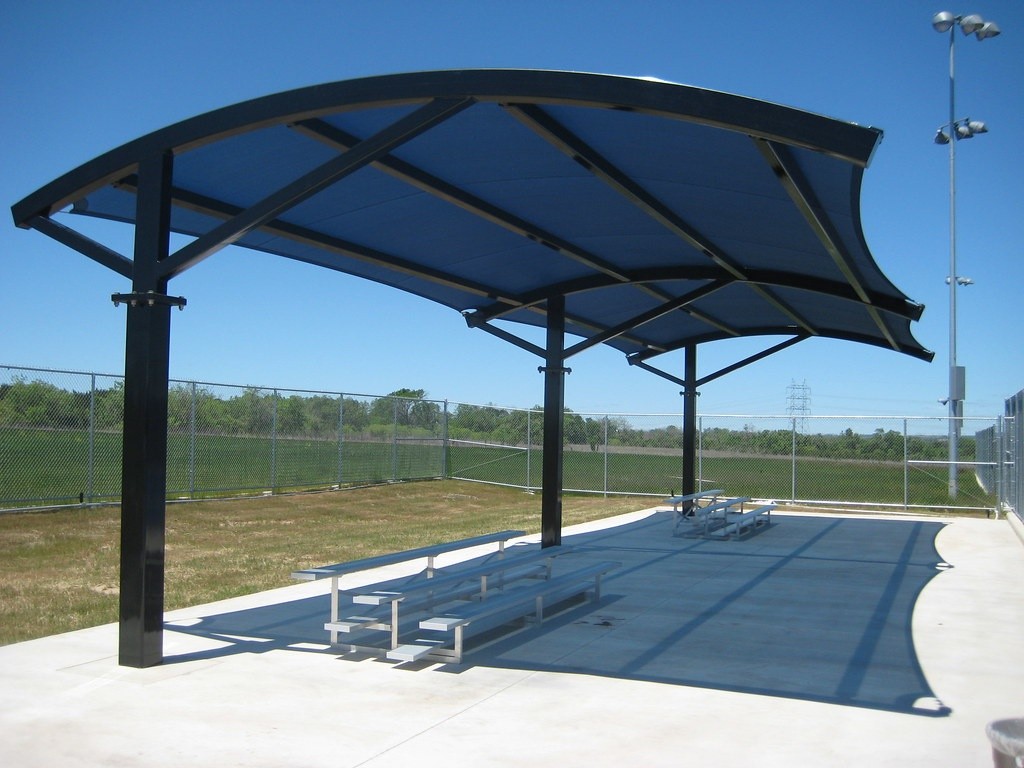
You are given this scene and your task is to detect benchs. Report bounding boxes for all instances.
[731,505,777,541]
[695,497,751,536]
[419,561,622,664]
[663,490,725,531]
[353,545,574,650]
[292,530,527,649]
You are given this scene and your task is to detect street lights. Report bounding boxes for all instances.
[932,11,1000,499]
